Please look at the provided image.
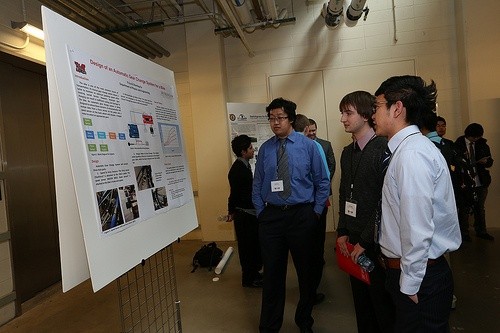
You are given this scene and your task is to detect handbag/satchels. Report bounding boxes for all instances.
[190,242,223,274]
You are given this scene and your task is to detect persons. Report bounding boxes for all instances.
[292,114,330,307]
[252,97,330,333]
[372,75,462,333]
[307,119,336,265]
[226,135,264,289]
[337,91,389,333]
[412,110,494,309]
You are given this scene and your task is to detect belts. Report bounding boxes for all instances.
[378,251,433,270]
[266,203,314,209]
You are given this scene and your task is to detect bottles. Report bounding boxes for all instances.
[357,255,375,272]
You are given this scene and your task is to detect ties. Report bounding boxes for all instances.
[469,142,478,179]
[277,138,292,200]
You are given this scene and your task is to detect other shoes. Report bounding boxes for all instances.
[476,233,494,241]
[463,235,473,242]
[300,327,314,333]
[242,272,264,287]
[313,292,325,306]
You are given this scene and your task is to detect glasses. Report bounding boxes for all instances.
[372,101,389,114]
[268,117,288,122]
